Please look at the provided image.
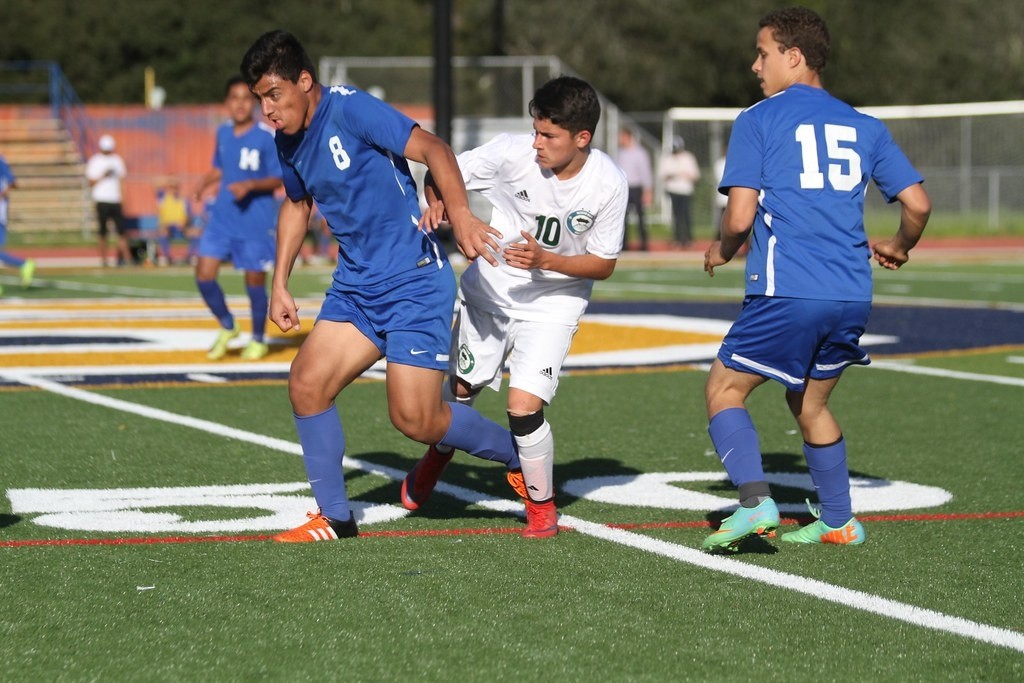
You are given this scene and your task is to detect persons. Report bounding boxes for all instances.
[191,77,284,360]
[713,143,729,242]
[85,136,137,268]
[662,136,702,246]
[0,158,36,297]
[704,5,931,552]
[242,30,526,542]
[153,177,198,267]
[400,76,629,540]
[617,128,654,252]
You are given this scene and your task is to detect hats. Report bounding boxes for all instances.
[99,136,113,150]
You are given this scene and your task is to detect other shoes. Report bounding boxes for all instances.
[20,260,34,287]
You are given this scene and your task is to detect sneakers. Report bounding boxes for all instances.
[507,471,526,498]
[702,498,780,551]
[523,499,558,537]
[274,509,359,543]
[241,340,269,359]
[400,447,456,510]
[781,498,866,545]
[208,325,238,360]
